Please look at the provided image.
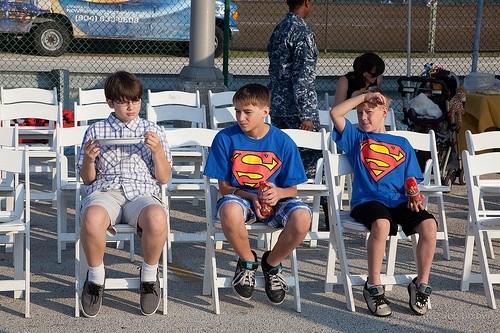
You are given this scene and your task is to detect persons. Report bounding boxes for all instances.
[75,70,174,319]
[402,79,447,130]
[201,83,313,306]
[267,0,330,231]
[329,89,438,317]
[334,52,385,106]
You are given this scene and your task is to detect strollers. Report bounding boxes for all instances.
[397,69,467,193]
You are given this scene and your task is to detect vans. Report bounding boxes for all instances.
[0,0,239,57]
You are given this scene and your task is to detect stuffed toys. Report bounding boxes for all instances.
[449,88,466,127]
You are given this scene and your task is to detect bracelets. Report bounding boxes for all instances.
[232,188,240,196]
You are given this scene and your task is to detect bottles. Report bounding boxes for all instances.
[405,178,423,208]
[258,181,274,218]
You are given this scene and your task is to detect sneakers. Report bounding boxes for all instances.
[261,251,288,305]
[81,270,106,318]
[232,250,259,300]
[407,277,432,315]
[363,276,392,318]
[138,266,161,316]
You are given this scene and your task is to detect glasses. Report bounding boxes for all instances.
[367,70,384,78]
[110,97,143,106]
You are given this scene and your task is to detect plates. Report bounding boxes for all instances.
[90,137,148,146]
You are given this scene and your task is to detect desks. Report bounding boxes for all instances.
[459,90,500,160]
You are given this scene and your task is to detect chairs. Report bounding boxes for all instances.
[0,87,500,318]
